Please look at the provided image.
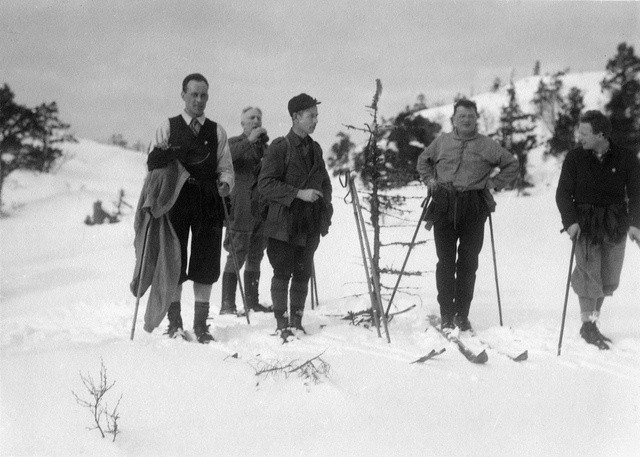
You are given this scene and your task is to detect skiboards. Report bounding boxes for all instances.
[424,309,528,364]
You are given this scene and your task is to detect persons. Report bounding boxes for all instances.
[415,99,520,336]
[555,109,640,352]
[144,73,235,345]
[220,106,272,315]
[257,93,333,343]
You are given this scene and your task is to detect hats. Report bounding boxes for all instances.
[288,94,321,114]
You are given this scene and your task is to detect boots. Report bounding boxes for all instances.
[243,270,273,316]
[166,300,187,342]
[219,272,241,320]
[191,300,215,344]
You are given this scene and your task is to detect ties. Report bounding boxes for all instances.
[190,119,199,136]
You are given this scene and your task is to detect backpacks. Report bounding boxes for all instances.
[249,135,292,221]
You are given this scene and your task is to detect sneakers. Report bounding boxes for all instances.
[578,321,608,351]
[440,312,456,338]
[453,313,472,330]
